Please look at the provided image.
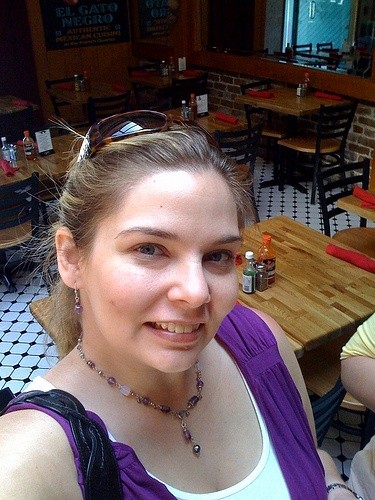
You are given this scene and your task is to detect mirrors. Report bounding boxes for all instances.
[176,0,375,102]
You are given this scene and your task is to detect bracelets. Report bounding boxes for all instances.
[327,483,363,500]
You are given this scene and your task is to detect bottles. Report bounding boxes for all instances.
[342,39,350,52]
[256,265,268,292]
[188,108,194,120]
[23,131,35,160]
[161,57,175,76]
[80,71,90,91]
[184,107,188,119]
[257,236,275,284]
[74,75,80,92]
[181,101,185,117]
[286,43,294,56]
[296,73,309,97]
[189,94,197,119]
[243,251,256,294]
[1,137,17,167]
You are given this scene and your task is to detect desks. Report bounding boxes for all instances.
[44,81,127,121]
[130,68,199,111]
[0,104,240,277]
[335,190,375,223]
[29,217,375,359]
[234,88,344,194]
[0,94,39,116]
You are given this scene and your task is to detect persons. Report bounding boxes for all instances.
[340,313,375,500]
[0,110,363,500]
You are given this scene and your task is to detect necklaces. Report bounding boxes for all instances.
[77,333,205,458]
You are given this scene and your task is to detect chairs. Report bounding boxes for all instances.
[0,66,375,500]
[256,42,372,79]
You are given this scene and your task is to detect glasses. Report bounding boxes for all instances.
[77,109,226,163]
